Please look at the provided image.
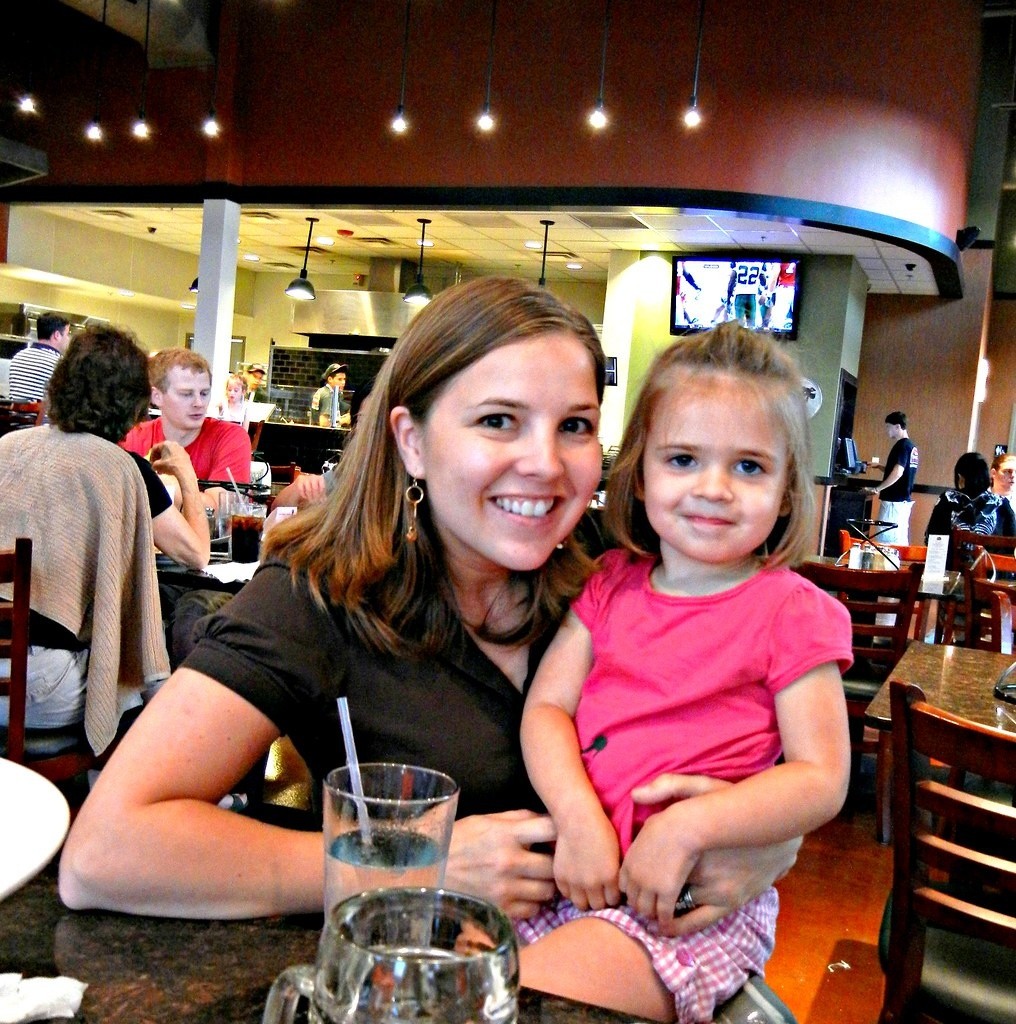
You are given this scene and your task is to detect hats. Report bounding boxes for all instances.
[325,363,347,378]
[247,363,266,374]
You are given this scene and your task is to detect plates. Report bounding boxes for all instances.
[0,757,71,901]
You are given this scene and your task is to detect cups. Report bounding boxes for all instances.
[262,888,519,1024]
[231,504,267,565]
[319,763,460,925]
[218,492,250,543]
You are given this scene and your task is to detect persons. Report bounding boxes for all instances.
[311,363,350,428]
[243,363,268,403]
[858,411,918,544]
[924,452,1016,647]
[118,347,252,660]
[214,375,249,434]
[520,320,855,1024]
[677,263,796,323]
[60,276,608,920]
[270,383,373,511]
[0,327,212,793]
[8,314,70,421]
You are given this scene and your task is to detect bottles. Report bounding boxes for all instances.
[205,507,216,541]
[848,542,900,571]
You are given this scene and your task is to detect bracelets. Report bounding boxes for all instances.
[676,890,695,910]
[875,487,878,491]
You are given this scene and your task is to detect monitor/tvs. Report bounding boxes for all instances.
[670,256,804,340]
[836,435,859,473]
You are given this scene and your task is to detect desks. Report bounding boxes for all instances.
[1,804,660,1024]
[136,477,282,592]
[863,640,1015,737]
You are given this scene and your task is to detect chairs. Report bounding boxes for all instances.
[0,396,1015,1024]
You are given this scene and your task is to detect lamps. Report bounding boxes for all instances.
[1,0,709,142]
[189,277,198,293]
[284,217,319,301]
[403,218,435,304]
